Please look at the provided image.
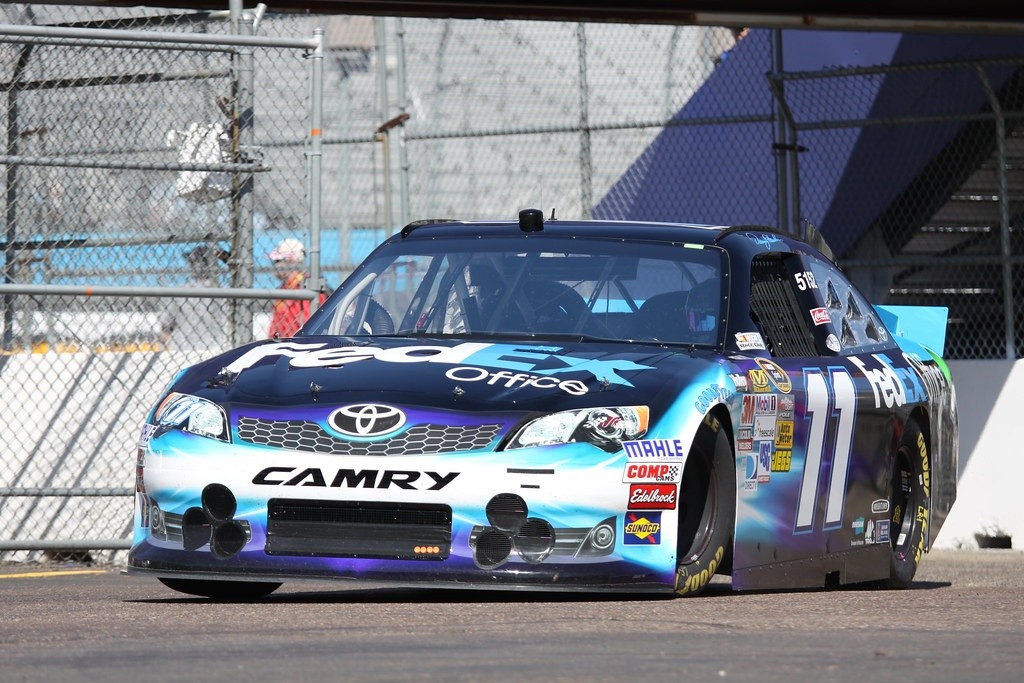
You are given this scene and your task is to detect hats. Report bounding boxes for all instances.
[181,246,214,267]
[269,238,305,262]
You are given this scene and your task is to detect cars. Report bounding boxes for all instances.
[126,208,959,600]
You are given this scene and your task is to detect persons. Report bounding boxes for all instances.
[267,238,325,339]
[683,278,722,346]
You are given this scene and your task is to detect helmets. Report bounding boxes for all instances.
[685,277,720,344]
